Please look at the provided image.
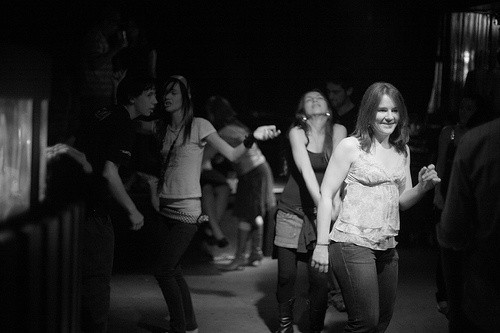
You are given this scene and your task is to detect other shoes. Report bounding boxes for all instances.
[161,316,171,329]
[437,300,447,313]
[228,255,244,269]
[201,231,216,246]
[217,238,229,248]
[248,251,262,264]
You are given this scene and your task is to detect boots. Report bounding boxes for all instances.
[273,296,295,333]
